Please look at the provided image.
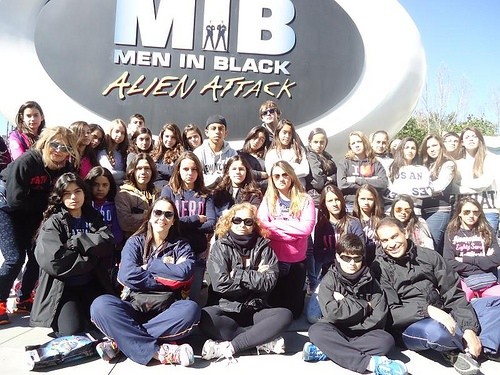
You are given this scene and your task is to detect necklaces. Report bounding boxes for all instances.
[232,185,239,188]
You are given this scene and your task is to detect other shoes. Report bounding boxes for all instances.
[438,350,479,375]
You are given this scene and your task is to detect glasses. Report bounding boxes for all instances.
[152,209,174,219]
[461,210,479,216]
[232,217,253,226]
[49,142,73,153]
[272,172,288,178]
[395,207,412,213]
[339,255,364,263]
[261,109,277,115]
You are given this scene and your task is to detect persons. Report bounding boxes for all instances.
[0,100,499,375]
[302,234,408,375]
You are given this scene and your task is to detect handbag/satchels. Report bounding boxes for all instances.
[131,287,175,314]
[464,271,497,290]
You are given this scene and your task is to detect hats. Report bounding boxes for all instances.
[205,114,226,128]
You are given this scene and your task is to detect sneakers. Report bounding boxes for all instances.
[303,342,328,361]
[256,335,284,356]
[96,339,121,360]
[13,297,34,313]
[373,356,408,375]
[0,303,9,323]
[159,344,194,367]
[202,338,236,363]
